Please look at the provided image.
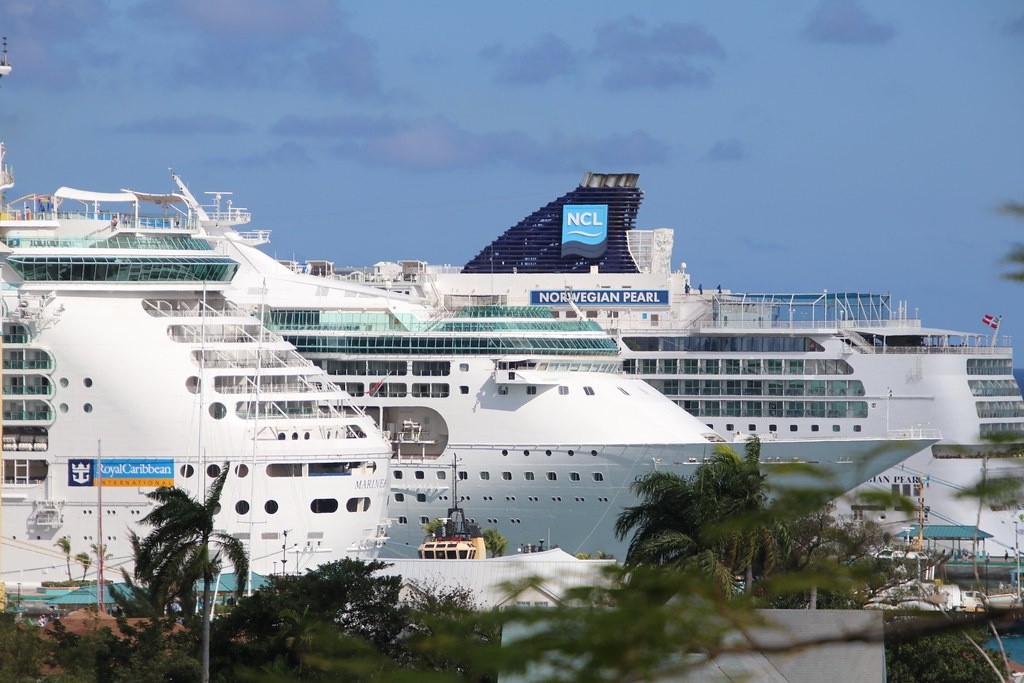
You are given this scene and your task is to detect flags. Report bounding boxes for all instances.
[982,313,1000,330]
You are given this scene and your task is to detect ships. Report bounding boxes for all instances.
[219,231,732,560]
[237,229,1024,575]
[2,166,392,591]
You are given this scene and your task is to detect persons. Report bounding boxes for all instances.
[997,581,1004,590]
[986,552,991,561]
[1014,581,1018,587]
[1004,550,1009,561]
[962,548,968,563]
[1006,581,1013,589]
[23,206,32,220]
[173,213,181,227]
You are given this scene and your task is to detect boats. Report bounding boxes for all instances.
[416,453,487,560]
[845,524,1023,579]
[869,581,1024,610]
